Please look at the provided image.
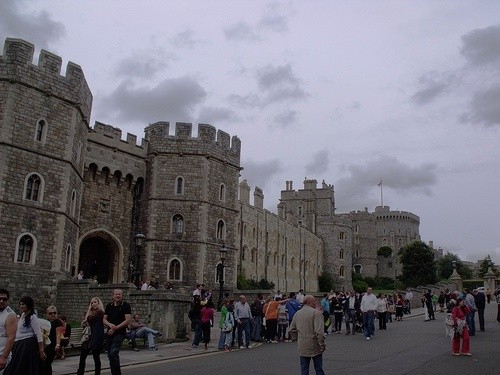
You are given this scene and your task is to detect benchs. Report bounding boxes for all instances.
[66,328,87,349]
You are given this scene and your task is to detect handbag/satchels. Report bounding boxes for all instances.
[222,324,233,333]
[263,317,266,326]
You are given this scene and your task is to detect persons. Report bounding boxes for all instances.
[188,282,214,349]
[452,297,472,355]
[218,288,306,352]
[138,279,157,291]
[129,313,163,350]
[361,287,378,340]
[321,289,413,335]
[125,320,141,352]
[421,286,500,335]
[92,276,98,286]
[77,271,84,280]
[290,294,327,375]
[0,289,72,375]
[75,297,105,375]
[103,289,132,375]
[164,282,175,291]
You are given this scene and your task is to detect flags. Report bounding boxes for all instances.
[378,180,382,186]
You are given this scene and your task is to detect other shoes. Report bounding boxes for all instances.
[463,352,472,356]
[158,332,163,336]
[60,356,65,359]
[128,339,132,344]
[133,348,140,351]
[452,352,461,356]
[54,356,57,359]
[149,347,158,351]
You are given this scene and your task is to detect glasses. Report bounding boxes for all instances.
[49,312,56,315]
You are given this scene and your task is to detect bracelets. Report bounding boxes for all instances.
[56,344,60,346]
[40,351,43,353]
